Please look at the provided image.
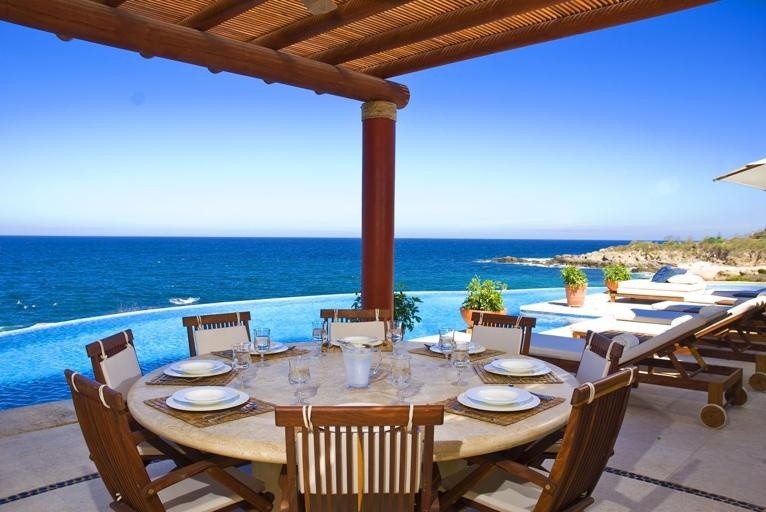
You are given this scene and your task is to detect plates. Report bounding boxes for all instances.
[327,335,384,349]
[452,359,553,415]
[429,342,486,355]
[164,357,250,414]
[246,336,289,355]
[317,402,400,433]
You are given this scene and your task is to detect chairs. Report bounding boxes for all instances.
[64,369,275,512]
[275,405,444,511]
[322,308,393,342]
[470,311,536,355]
[519,263,766,431]
[439,365,637,512]
[523,328,623,467]
[86,331,189,475]
[183,310,251,357]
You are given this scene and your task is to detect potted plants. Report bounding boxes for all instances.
[460,272,508,333]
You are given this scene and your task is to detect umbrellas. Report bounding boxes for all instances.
[713,159,766,192]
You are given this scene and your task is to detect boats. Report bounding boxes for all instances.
[168,296,201,306]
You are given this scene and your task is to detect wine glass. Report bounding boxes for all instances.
[232,342,253,391]
[252,327,270,368]
[386,319,414,406]
[286,357,310,407]
[312,320,329,358]
[439,327,470,387]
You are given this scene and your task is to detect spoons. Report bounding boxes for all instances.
[207,402,257,422]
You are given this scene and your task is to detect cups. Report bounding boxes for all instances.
[337,335,383,389]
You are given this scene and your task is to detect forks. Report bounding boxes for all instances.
[159,376,203,382]
[287,346,296,350]
